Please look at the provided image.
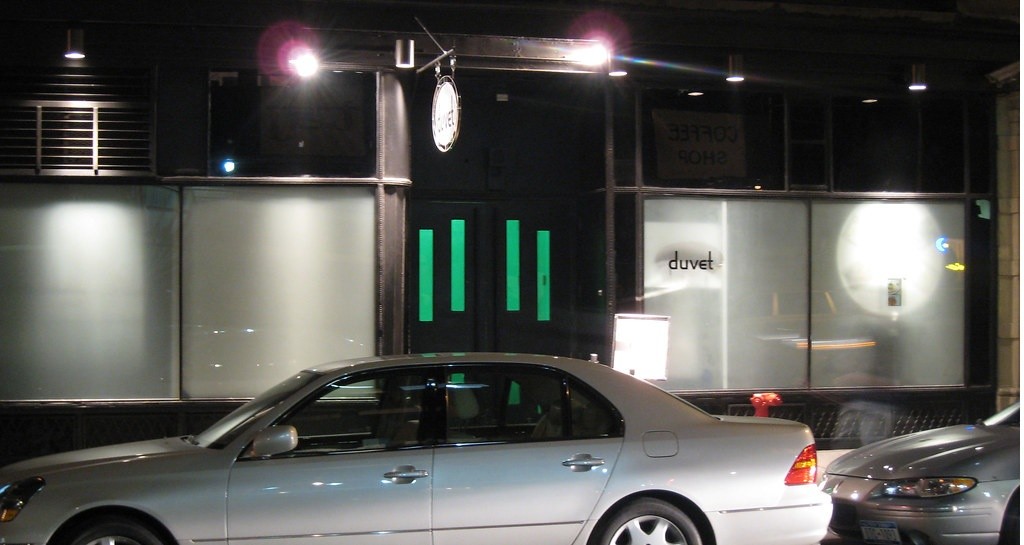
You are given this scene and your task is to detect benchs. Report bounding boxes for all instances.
[530,400,604,440]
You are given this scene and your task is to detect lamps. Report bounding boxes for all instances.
[726,53,745,82]
[395,40,414,68]
[63,28,87,59]
[608,53,627,76]
[909,63,927,91]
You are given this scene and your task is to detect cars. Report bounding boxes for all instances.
[818,397,1020,545]
[0,349,835,545]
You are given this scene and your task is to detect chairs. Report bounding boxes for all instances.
[448,385,479,443]
